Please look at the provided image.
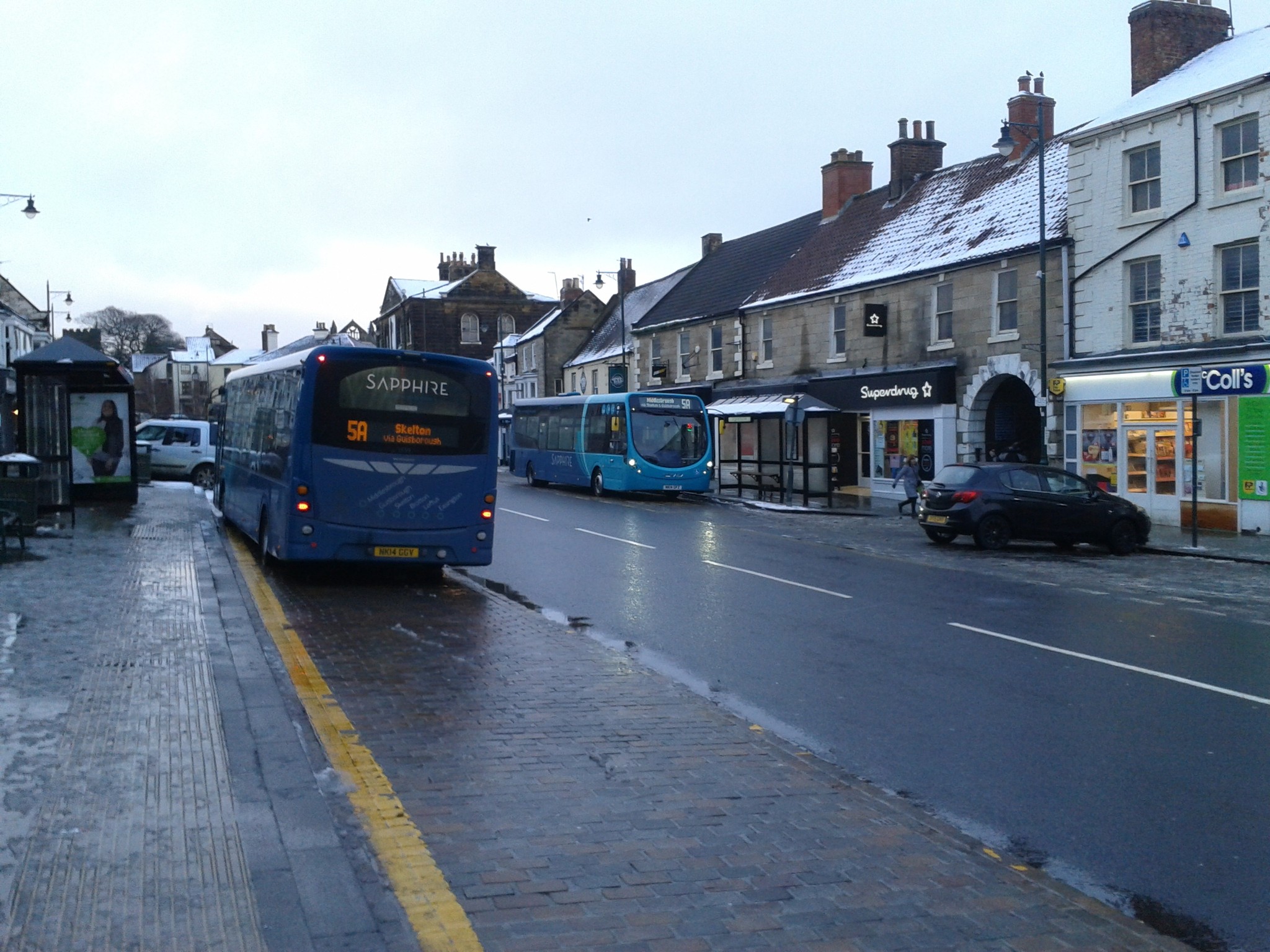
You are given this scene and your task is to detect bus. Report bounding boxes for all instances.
[508,392,728,497]
[202,345,499,580]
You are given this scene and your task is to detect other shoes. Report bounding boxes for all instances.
[898,503,902,512]
[911,513,918,518]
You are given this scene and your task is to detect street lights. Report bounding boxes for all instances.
[594,271,627,392]
[47,290,74,332]
[992,119,1048,464]
[50,309,73,343]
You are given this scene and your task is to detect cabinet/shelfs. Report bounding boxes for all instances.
[1126,434,1194,494]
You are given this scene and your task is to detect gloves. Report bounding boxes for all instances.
[917,480,921,487]
[892,483,895,488]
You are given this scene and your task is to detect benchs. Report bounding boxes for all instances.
[730,470,780,484]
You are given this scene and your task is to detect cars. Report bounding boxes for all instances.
[917,463,1152,557]
[134,419,217,490]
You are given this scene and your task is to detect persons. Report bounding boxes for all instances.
[87,400,123,477]
[989,442,1026,463]
[892,454,921,517]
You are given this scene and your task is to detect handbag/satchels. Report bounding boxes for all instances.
[917,486,924,496]
